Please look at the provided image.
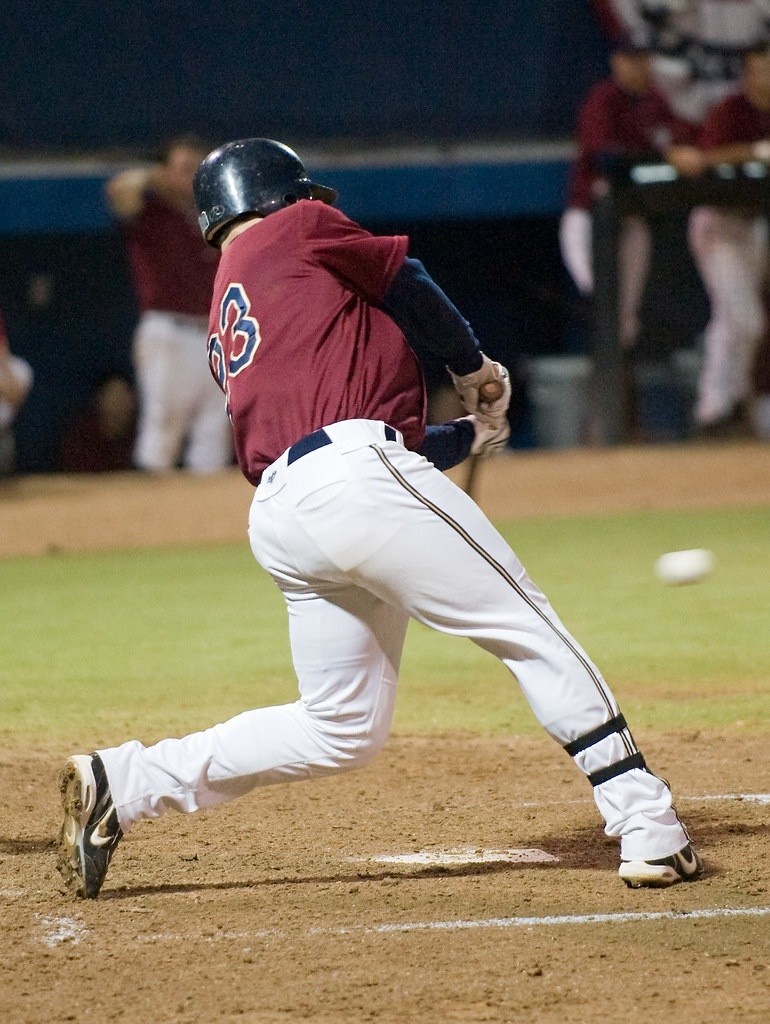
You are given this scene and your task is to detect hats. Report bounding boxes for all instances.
[614,31,653,57]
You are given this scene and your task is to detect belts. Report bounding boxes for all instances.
[288,426,396,464]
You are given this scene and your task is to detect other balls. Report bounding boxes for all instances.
[661,548,710,584]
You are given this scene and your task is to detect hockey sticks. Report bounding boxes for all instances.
[459,380,509,498]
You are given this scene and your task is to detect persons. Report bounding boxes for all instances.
[57,137,700,896]
[0,0,770,474]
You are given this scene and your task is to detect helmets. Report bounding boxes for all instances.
[194,137,337,250]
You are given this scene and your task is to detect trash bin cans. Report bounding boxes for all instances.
[532,355,623,449]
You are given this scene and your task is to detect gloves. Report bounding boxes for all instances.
[447,351,512,427]
[452,414,511,455]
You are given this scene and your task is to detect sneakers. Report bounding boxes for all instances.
[58,750,123,901]
[618,843,703,886]
